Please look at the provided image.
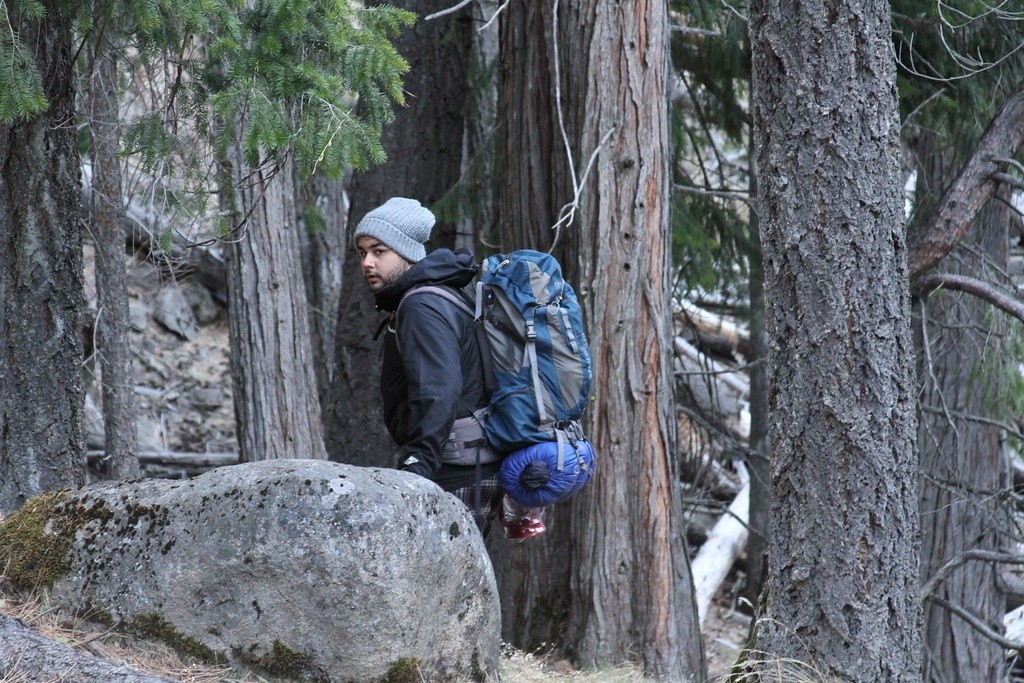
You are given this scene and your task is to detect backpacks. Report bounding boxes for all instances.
[394,250,592,456]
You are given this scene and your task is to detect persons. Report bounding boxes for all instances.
[352,197,503,551]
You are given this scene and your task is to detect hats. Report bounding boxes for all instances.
[352,196,437,264]
